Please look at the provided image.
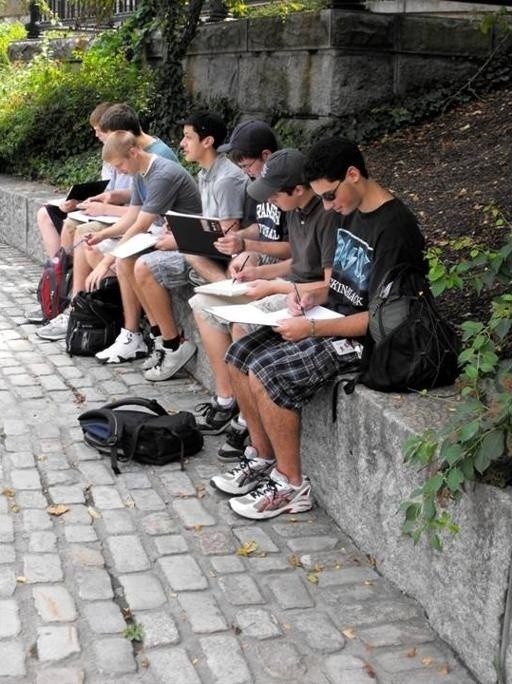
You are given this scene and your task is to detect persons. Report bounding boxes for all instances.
[118,110,256,381]
[23,101,131,322]
[83,129,202,364]
[34,102,181,341]
[210,136,462,521]
[187,147,339,462]
[184,119,290,283]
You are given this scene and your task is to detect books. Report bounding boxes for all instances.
[192,276,291,297]
[165,209,239,260]
[200,294,345,327]
[47,180,111,208]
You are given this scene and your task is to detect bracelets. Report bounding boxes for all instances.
[310,319,315,338]
[239,239,246,253]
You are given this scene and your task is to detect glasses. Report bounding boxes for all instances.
[313,180,341,200]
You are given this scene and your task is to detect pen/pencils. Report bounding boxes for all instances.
[85,234,90,241]
[232,255,249,284]
[225,222,236,234]
[292,280,306,317]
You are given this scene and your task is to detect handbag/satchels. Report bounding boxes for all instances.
[36,246,72,320]
[65,276,124,356]
[359,262,458,392]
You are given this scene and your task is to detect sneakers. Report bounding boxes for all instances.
[210,447,278,495]
[142,333,197,382]
[228,468,313,520]
[24,304,44,322]
[217,415,252,462]
[34,308,71,340]
[195,395,241,435]
[94,327,149,363]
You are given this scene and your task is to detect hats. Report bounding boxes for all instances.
[176,111,227,141]
[216,121,277,153]
[246,149,308,202]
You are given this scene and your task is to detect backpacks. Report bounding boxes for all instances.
[78,398,203,475]
